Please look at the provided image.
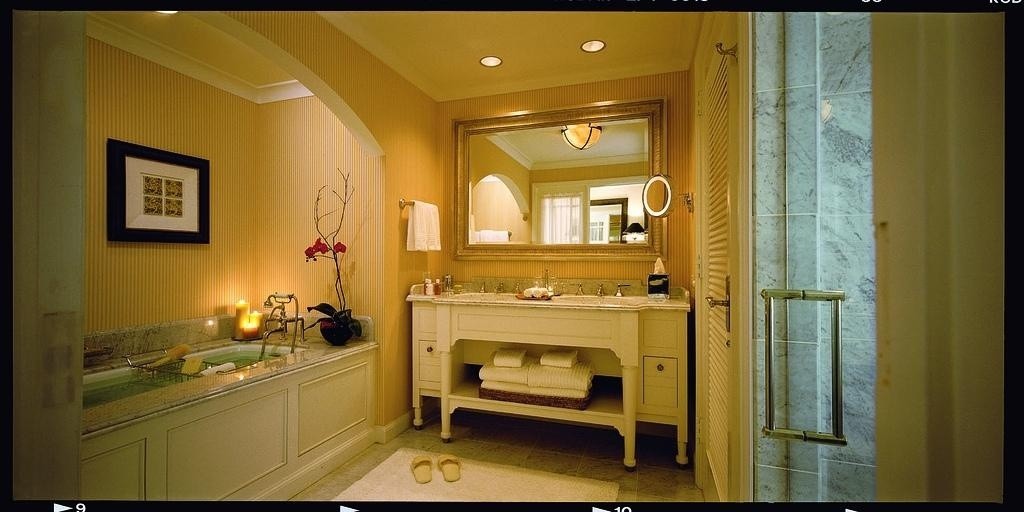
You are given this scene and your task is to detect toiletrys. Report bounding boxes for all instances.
[423,274,451,295]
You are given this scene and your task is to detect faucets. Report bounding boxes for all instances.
[263,327,283,339]
[494,281,505,293]
[596,283,606,296]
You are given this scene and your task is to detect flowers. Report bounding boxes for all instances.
[302,164,362,336]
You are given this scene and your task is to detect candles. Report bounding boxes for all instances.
[235,300,250,338]
[243,323,258,338]
[250,311,265,338]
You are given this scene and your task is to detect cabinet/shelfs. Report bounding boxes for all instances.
[406,298,690,471]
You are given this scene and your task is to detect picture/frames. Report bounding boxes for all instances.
[103,134,211,247]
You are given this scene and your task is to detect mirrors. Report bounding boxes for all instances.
[449,93,670,263]
[642,172,695,216]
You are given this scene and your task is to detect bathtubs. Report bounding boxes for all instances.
[83,343,307,411]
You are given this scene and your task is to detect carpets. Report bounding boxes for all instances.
[320,445,619,503]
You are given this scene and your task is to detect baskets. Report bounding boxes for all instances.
[122,349,262,385]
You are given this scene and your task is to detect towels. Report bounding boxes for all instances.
[199,362,236,376]
[533,288,548,297]
[474,229,510,246]
[407,201,441,254]
[523,287,537,297]
[477,347,597,411]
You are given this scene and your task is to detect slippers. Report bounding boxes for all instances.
[412,456,431,484]
[438,455,460,481]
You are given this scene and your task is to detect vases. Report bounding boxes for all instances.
[319,320,350,345]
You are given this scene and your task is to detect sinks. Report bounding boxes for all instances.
[453,293,520,303]
[553,297,632,305]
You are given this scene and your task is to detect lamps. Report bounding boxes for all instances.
[556,120,601,151]
